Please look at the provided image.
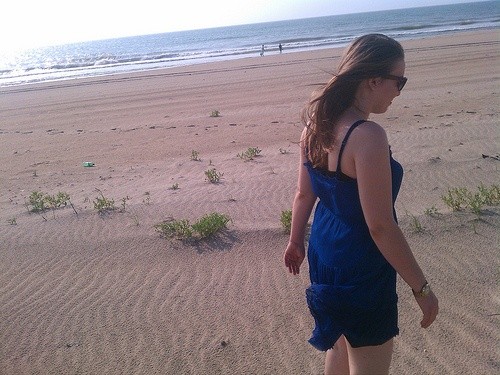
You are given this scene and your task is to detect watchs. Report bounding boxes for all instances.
[411,280,431,297]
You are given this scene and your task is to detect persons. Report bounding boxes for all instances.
[284,33,440,375]
[279,43,283,54]
[260,45,267,56]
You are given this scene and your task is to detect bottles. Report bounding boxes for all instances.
[83,162,94,167]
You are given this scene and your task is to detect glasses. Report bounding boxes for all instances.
[377,74,408,91]
